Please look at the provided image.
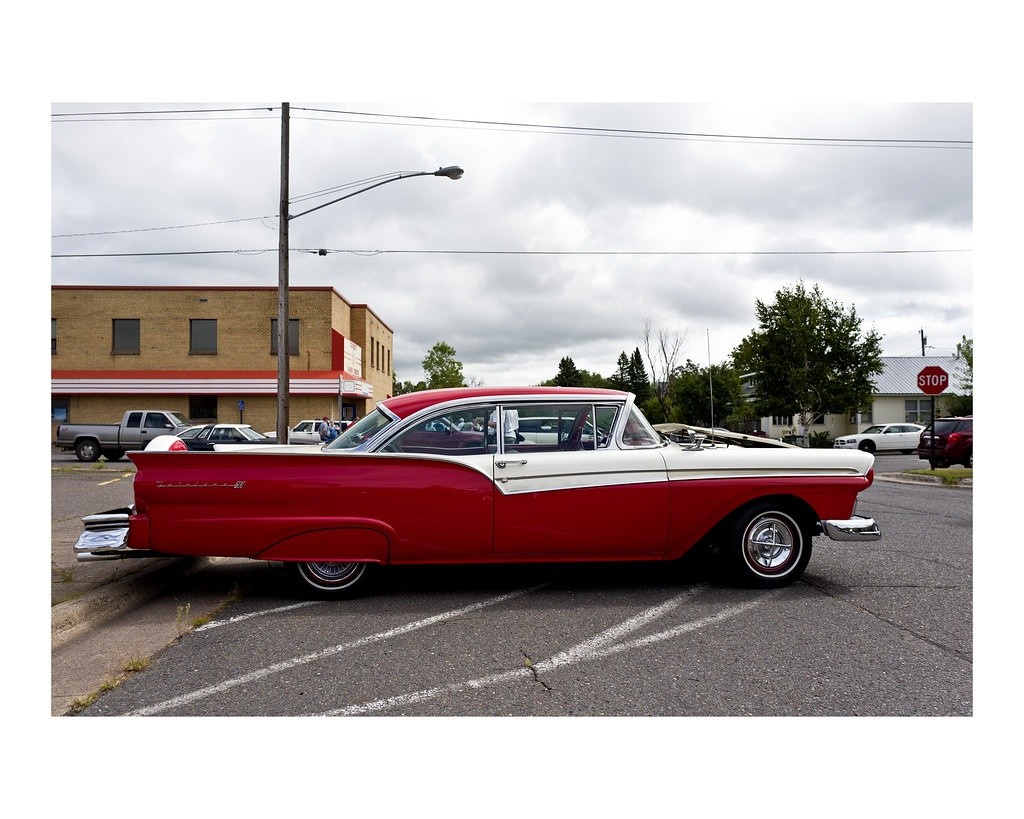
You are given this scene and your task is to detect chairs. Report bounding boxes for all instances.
[221,429,232,440]
[208,428,223,440]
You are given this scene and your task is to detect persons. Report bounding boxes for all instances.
[488,409,519,445]
[319,417,360,444]
[457,417,484,432]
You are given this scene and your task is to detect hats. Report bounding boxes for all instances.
[322,416,329,420]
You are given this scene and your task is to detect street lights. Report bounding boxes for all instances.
[274,164,464,442]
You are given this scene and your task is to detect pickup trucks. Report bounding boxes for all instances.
[56,410,195,463]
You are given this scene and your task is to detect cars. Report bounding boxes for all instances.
[69,386,886,605]
[833,423,925,454]
[916,415,973,468]
[264,419,353,444]
[176,424,274,450]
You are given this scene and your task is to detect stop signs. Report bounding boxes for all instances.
[917,365,950,396]
